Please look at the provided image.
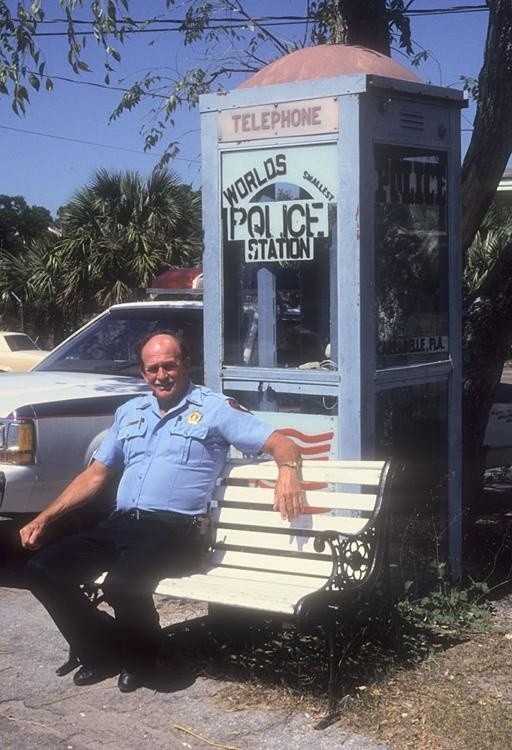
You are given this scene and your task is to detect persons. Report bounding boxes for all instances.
[20,329,309,695]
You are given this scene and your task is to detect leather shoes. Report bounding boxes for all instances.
[118,666,152,692]
[73,663,120,686]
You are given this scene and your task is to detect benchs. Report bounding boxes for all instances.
[55,452,409,715]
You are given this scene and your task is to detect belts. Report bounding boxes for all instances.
[110,509,205,529]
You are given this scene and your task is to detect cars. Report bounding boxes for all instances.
[304,223,450,458]
[1,266,299,572]
[1,331,81,373]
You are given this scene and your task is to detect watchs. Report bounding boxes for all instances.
[276,460,304,474]
